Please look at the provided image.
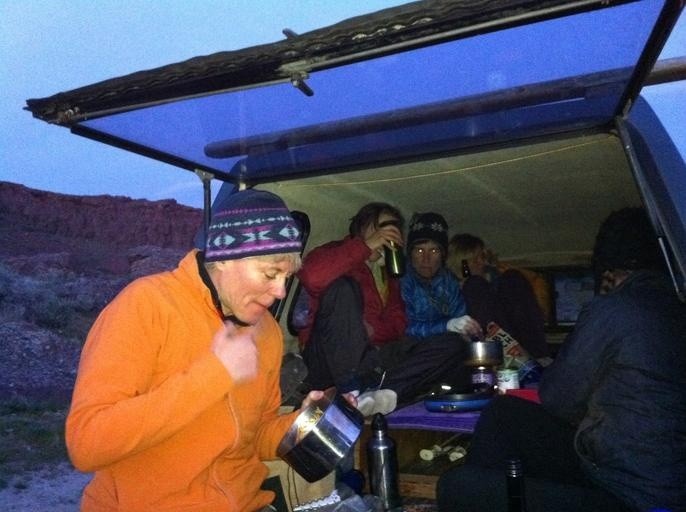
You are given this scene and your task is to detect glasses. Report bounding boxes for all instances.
[413,247,441,255]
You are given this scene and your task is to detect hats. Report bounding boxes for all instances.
[205,188,303,262]
[407,212,449,258]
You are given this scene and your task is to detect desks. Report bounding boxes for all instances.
[353,377,540,500]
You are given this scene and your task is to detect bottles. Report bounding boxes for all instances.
[496,366,520,394]
[460,258,473,278]
[471,365,492,394]
[366,412,400,508]
[379,220,406,278]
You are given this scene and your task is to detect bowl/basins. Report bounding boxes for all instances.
[277,386,365,484]
[462,341,502,368]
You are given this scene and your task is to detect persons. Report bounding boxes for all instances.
[298,203,553,416]
[438,207,686,512]
[65,190,357,512]
